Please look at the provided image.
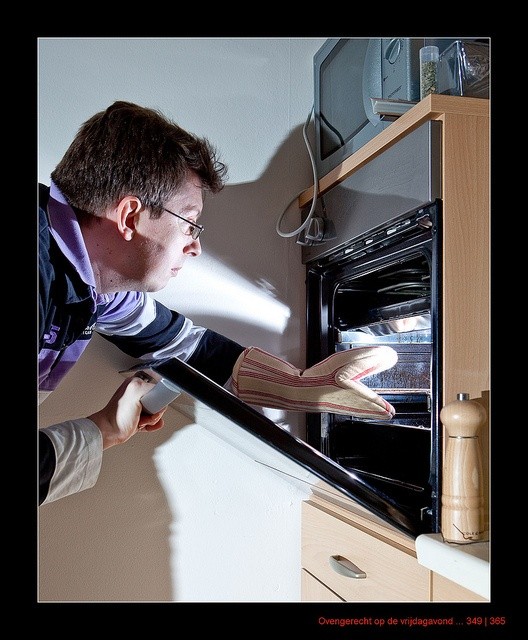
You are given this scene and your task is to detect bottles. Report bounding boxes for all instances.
[439,391,487,546]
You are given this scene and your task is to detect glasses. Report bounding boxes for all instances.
[164,208,204,240]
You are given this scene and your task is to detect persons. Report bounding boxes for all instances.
[38,102,399,507]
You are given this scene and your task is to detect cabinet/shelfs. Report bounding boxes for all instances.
[431,571,492,603]
[301,498,432,602]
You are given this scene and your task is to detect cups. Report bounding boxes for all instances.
[419,45,440,101]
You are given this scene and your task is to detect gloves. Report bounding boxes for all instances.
[230,345,398,420]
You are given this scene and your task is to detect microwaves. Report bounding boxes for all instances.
[118,92,488,546]
[313,35,474,180]
